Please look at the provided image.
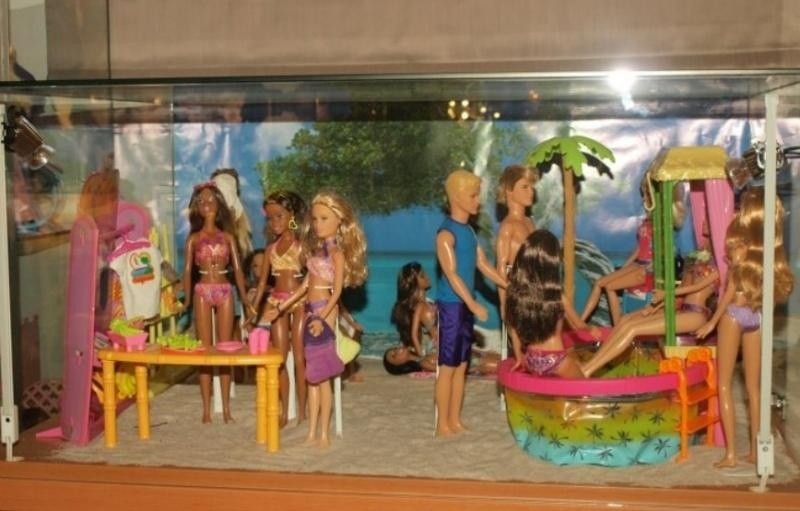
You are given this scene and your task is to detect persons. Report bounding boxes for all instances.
[384,263,501,375]
[435,170,512,437]
[4,130,795,471]
[695,184,792,469]
[186,169,369,448]
[497,167,721,376]
